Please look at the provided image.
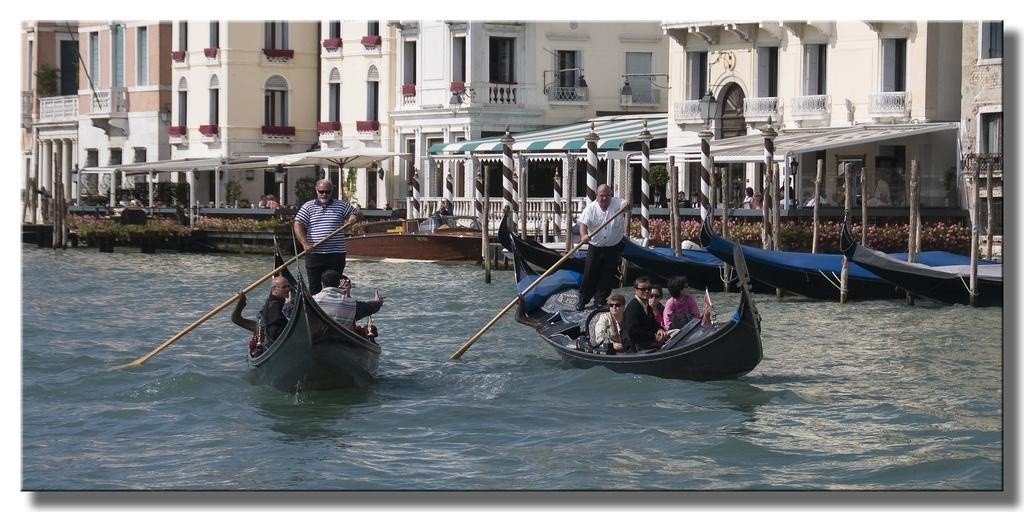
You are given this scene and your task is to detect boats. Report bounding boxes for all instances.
[511,235,764,382]
[247,235,382,390]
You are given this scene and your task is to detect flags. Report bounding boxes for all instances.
[701,290,711,327]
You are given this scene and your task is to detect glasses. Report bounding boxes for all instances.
[608,303,622,307]
[277,284,290,288]
[317,189,331,194]
[755,194,761,197]
[649,294,661,299]
[635,287,651,291]
[683,285,689,288]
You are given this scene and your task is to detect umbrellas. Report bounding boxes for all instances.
[268,146,412,201]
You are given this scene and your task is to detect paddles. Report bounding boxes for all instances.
[448,209,623,358]
[108,222,352,370]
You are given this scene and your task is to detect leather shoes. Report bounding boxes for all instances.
[593,300,607,308]
[576,298,585,311]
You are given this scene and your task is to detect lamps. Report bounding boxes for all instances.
[544,69,588,96]
[449,89,471,117]
[698,50,732,131]
[71,164,80,183]
[272,164,286,184]
[790,158,799,175]
[620,72,671,106]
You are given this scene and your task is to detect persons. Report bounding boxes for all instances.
[119,194,131,207]
[292,179,361,294]
[576,184,632,310]
[677,192,691,209]
[231,275,383,356]
[433,206,448,228]
[130,194,143,207]
[266,194,279,208]
[258,195,266,208]
[596,276,700,355]
[385,203,392,210]
[743,169,893,209]
